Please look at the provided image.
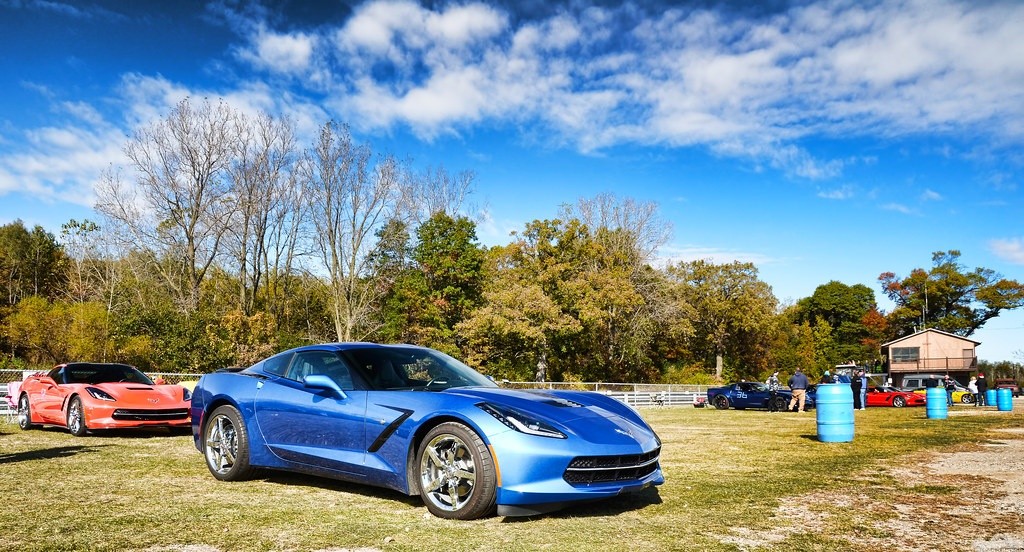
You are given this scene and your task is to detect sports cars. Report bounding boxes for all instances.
[190,342,666,522]
[865,385,926,408]
[913,385,983,404]
[707,382,815,413]
[16,362,196,437]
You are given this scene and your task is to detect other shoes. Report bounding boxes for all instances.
[854,408,859,410]
[860,408,865,410]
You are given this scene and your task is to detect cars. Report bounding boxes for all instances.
[806,384,815,402]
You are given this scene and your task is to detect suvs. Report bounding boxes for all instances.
[994,378,1019,397]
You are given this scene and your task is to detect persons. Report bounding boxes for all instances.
[850,369,867,410]
[944,374,956,407]
[840,359,855,365]
[875,359,879,373]
[926,374,937,388]
[821,371,831,384]
[968,376,978,406]
[787,367,808,413]
[766,369,779,412]
[864,360,869,373]
[975,373,988,406]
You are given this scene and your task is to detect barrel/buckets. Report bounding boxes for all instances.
[816,383,855,442]
[984,389,997,406]
[926,387,947,418]
[997,388,1013,411]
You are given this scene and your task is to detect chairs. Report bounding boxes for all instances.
[303,356,325,375]
[693,394,708,408]
[5,382,23,424]
[650,393,666,408]
[373,363,409,389]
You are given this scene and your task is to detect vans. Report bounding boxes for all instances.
[902,373,971,392]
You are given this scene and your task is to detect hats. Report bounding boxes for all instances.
[978,373,985,379]
